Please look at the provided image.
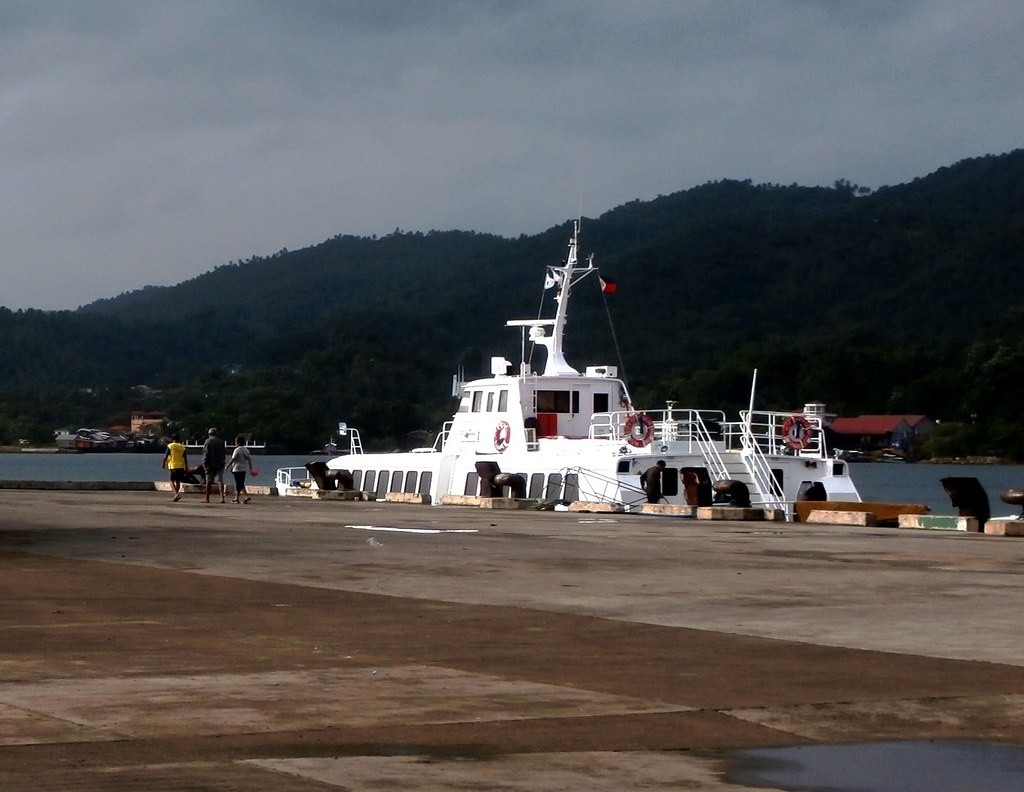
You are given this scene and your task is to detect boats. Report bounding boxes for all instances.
[276,220,863,518]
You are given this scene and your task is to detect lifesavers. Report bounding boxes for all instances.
[494,421,509,453]
[623,413,655,448]
[781,415,813,450]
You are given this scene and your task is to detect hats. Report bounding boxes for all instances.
[249,467,261,477]
[172,434,178,439]
[208,428,217,434]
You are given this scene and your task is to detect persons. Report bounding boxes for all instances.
[225,436,253,504]
[640,460,666,503]
[161,433,188,502]
[200,428,225,502]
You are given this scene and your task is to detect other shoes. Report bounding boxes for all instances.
[240,497,251,504]
[171,494,182,502]
[229,499,239,503]
[200,499,209,503]
[218,499,225,504]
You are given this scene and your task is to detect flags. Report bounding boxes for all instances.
[604,283,616,293]
